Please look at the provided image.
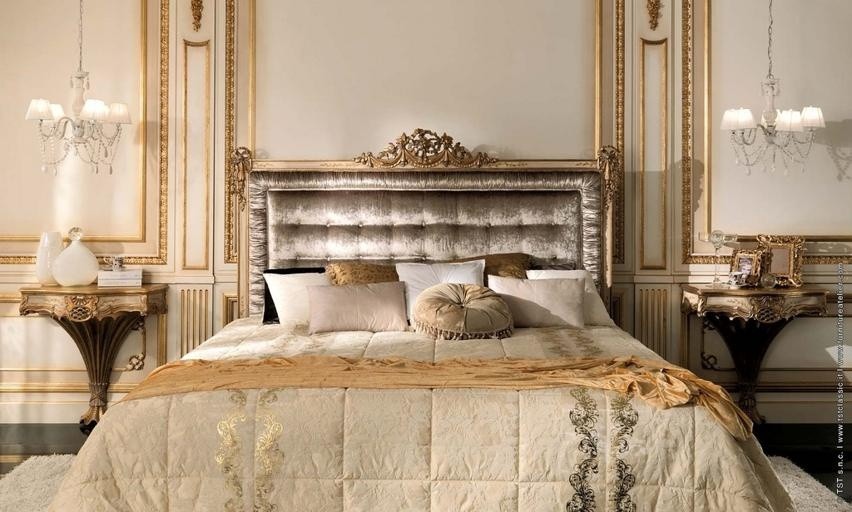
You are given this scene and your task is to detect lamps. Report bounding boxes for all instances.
[25,2,134,177]
[714,0,825,182]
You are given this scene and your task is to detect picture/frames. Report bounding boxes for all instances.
[729,246,763,283]
[755,235,810,290]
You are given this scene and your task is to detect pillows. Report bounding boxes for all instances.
[525,267,615,329]
[259,266,325,324]
[303,279,411,333]
[393,258,488,322]
[486,272,587,331]
[260,271,332,326]
[324,260,399,287]
[409,281,516,342]
[447,251,533,282]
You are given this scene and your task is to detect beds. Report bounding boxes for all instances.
[58,127,795,510]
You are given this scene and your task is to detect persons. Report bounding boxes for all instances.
[739,258,752,275]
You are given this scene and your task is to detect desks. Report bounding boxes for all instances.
[676,280,830,457]
[18,282,167,437]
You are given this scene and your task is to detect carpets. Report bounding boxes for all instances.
[764,452,851,512]
[0,452,78,512]
